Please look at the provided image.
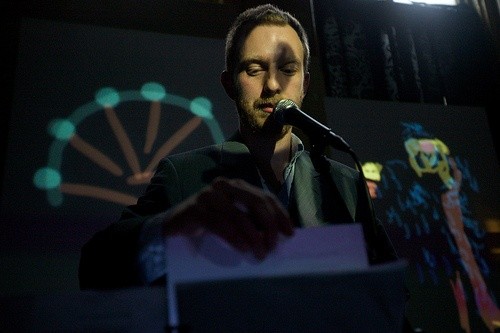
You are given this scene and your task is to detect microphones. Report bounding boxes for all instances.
[273,98,351,153]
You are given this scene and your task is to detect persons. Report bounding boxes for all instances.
[94,2,399,282]
